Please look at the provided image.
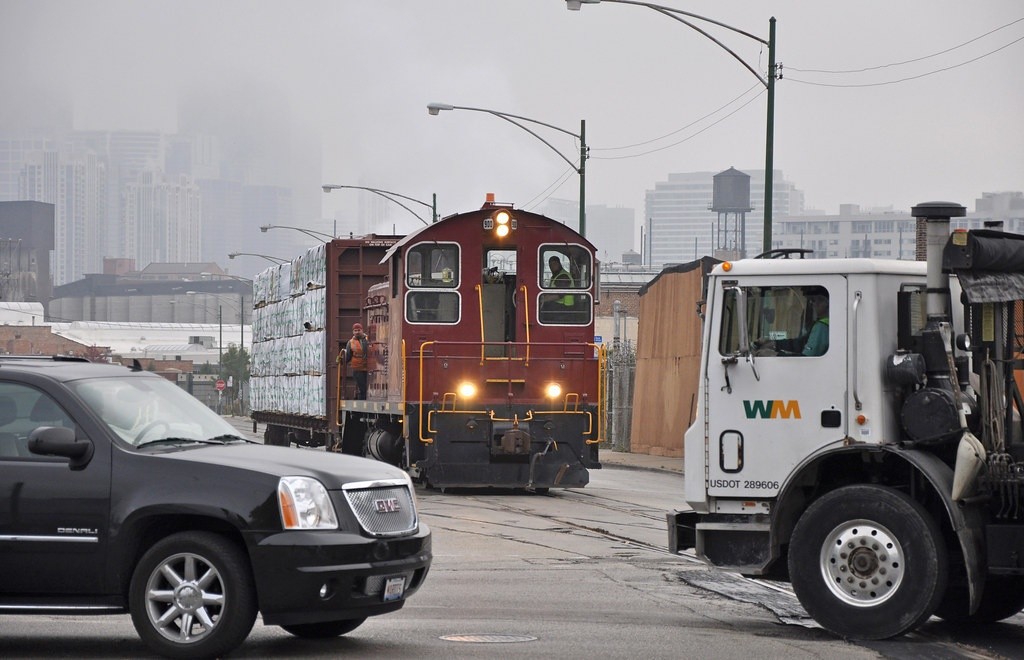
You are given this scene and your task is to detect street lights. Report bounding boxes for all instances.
[567,0,776,260]
[322,183,437,227]
[427,103,586,240]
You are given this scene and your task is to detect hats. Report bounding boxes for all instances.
[352,323,363,329]
[801,286,829,299]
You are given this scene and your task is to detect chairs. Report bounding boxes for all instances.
[0,395,31,457]
[27,393,75,457]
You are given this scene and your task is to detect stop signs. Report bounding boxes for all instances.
[214,380,226,391]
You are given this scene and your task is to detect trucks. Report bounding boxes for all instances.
[665,197,1024,641]
[248,193,609,497]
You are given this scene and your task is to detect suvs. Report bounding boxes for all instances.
[0,354,433,660]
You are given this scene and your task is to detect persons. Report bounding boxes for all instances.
[336,323,368,400]
[541,256,575,309]
[761,293,829,356]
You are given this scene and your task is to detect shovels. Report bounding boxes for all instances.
[938,320,986,502]
